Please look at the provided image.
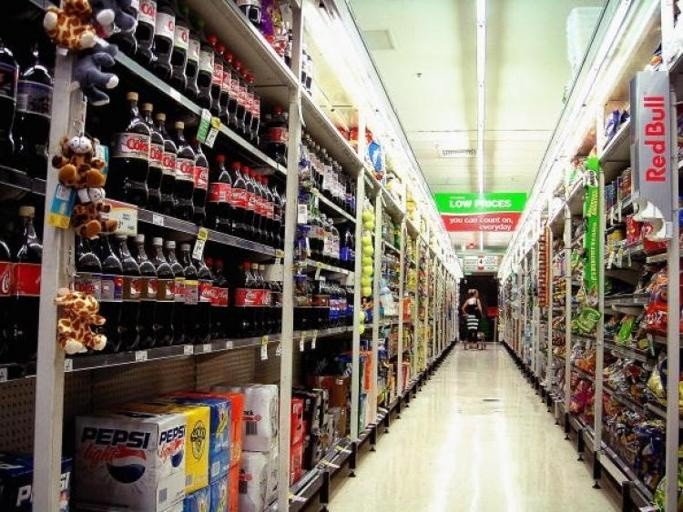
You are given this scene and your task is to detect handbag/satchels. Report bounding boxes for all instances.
[474,307,482,319]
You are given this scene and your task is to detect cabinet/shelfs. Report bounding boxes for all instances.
[66,0,300,511]
[1,1,71,511]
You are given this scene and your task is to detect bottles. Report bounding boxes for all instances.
[235,1,314,96]
[0,38,21,169]
[294,192,356,270]
[0,238,14,366]
[15,43,54,180]
[107,91,288,251]
[295,274,354,330]
[75,230,282,354]
[111,0,261,147]
[11,205,42,362]
[298,128,357,218]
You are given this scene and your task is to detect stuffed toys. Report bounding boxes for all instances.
[52,135,104,188]
[90,1,140,32]
[56,288,107,355]
[68,44,119,106]
[73,187,118,240]
[44,0,96,50]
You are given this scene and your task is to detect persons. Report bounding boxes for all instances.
[461,288,485,349]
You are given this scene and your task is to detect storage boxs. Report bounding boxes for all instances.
[0,449,75,512]
[72,375,357,511]
